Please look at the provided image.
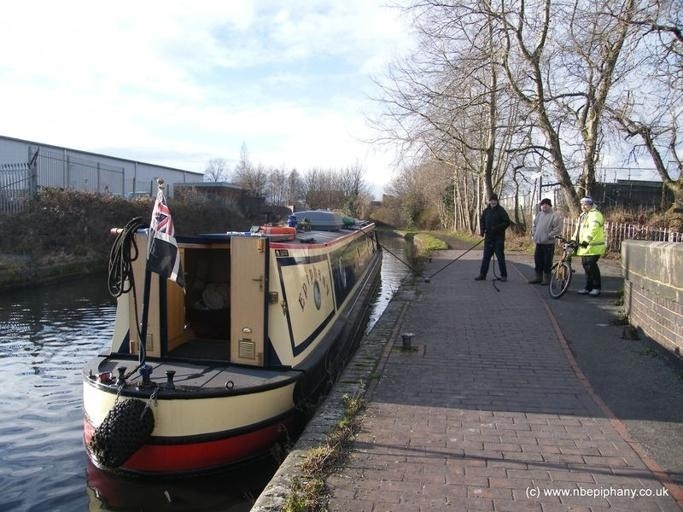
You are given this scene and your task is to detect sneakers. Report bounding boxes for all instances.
[578,289,589,293]
[589,288,600,295]
[502,272,507,281]
[474,272,487,280]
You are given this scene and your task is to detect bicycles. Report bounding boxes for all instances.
[547,234,588,299]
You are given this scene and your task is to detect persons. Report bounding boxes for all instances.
[528,198,561,286]
[569,196,606,296]
[474,193,510,282]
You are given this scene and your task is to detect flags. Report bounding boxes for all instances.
[145,187,187,295]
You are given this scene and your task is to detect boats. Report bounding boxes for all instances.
[80,449,255,511]
[80,178,385,480]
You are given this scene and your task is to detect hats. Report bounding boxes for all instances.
[489,194,498,201]
[539,199,552,205]
[580,196,592,204]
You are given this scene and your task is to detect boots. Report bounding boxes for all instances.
[541,271,550,285]
[528,272,543,283]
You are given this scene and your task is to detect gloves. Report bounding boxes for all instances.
[582,241,588,248]
